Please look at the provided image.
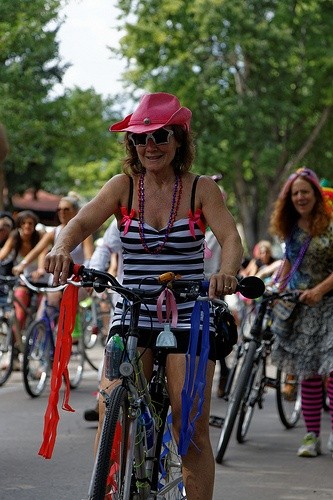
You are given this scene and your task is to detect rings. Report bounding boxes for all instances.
[224,285,231,290]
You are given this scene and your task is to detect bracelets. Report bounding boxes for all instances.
[138,172,184,254]
[22,261,28,267]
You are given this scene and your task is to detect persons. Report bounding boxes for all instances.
[265,169,333,457]
[0,93,328,500]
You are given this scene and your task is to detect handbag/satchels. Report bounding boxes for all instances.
[270,300,300,340]
[212,302,238,360]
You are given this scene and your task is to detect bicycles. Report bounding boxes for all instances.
[46,262,266,500]
[1,269,116,398]
[205,285,333,462]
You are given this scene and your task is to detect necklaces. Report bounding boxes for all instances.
[274,234,310,291]
[141,172,178,251]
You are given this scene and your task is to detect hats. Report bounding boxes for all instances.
[109,92,192,133]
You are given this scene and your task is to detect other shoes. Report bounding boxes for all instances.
[83,409,100,421]
[30,367,50,379]
[1,358,21,370]
[217,368,230,397]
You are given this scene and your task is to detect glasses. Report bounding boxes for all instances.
[128,127,174,147]
[57,207,69,213]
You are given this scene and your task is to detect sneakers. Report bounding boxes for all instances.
[298,432,321,457]
[328,432,333,452]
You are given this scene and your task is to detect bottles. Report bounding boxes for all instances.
[71,309,82,337]
[104,334,123,380]
